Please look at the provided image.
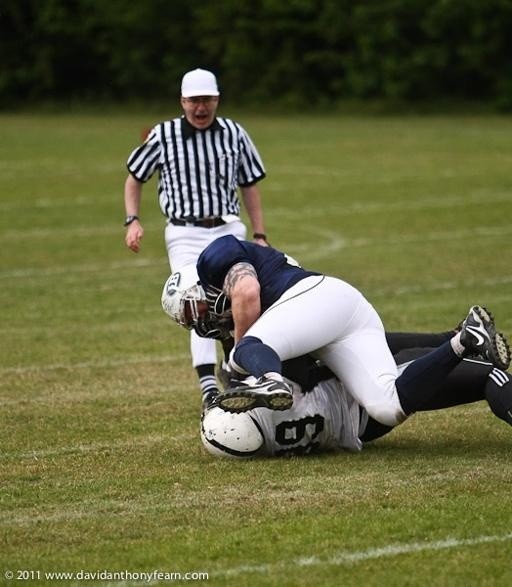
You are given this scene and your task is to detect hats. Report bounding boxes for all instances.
[181,68,218,98]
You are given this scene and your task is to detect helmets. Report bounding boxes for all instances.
[159,266,208,329]
[199,405,265,460]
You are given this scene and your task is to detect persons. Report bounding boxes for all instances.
[124,68,271,405]
[192,309,512,459]
[160,235,511,427]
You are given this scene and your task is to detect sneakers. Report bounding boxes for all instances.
[202,390,221,410]
[459,302,511,370]
[217,380,294,414]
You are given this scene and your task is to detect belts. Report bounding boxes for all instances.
[171,215,235,228]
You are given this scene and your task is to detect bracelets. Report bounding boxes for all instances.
[253,234,266,241]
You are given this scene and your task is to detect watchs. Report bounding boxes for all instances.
[124,216,138,226]
